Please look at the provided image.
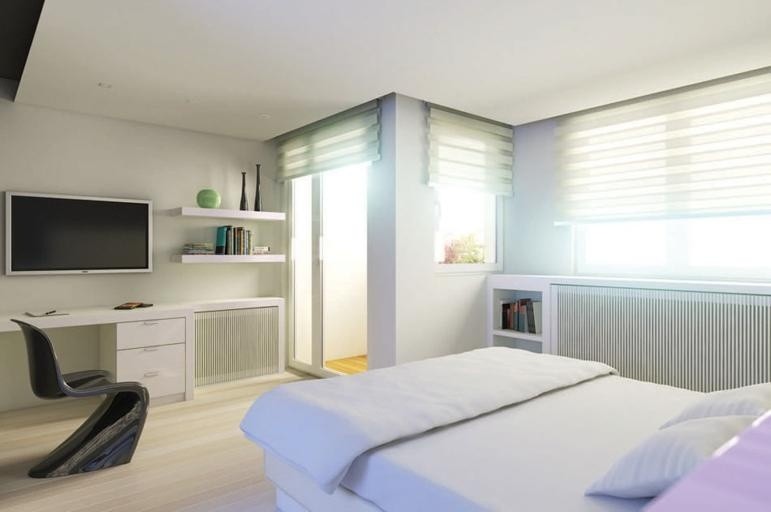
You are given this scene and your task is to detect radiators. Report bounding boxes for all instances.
[193,305,278,386]
[557,287,771,392]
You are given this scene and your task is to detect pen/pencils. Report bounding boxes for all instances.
[44,310,55,314]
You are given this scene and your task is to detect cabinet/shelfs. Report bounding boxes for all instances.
[489,276,551,354]
[2,302,194,407]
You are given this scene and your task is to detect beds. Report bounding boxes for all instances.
[240,347,771,512]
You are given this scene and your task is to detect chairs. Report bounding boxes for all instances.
[11,318,149,478]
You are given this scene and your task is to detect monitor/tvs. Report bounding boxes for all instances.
[6,191,153,276]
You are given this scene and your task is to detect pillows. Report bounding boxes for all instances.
[585,414,756,498]
[660,382,771,427]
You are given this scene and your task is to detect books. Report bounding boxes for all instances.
[216,225,250,258]
[183,243,214,255]
[500,297,541,335]
